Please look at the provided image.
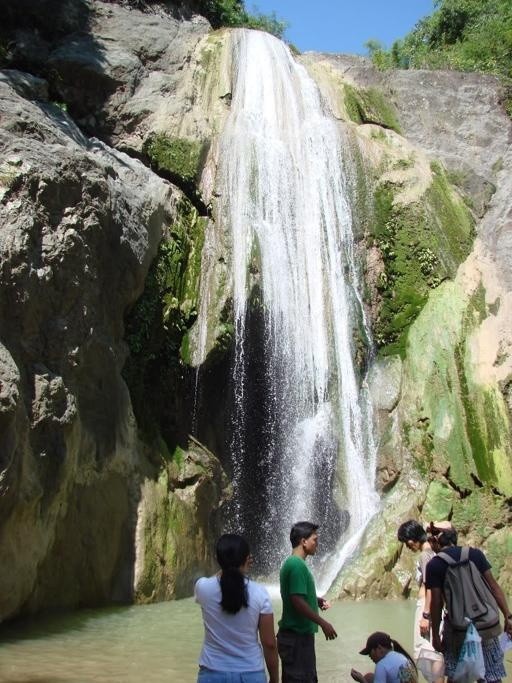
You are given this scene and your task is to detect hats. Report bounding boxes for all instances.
[356,630,391,655]
[421,526,435,542]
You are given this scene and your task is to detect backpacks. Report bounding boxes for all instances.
[431,544,504,645]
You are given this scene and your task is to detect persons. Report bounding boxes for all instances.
[277,521,338,682]
[398,518,446,682]
[192,532,280,682]
[427,519,511,682]
[351,630,419,682]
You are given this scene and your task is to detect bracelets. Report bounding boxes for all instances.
[422,610,431,618]
